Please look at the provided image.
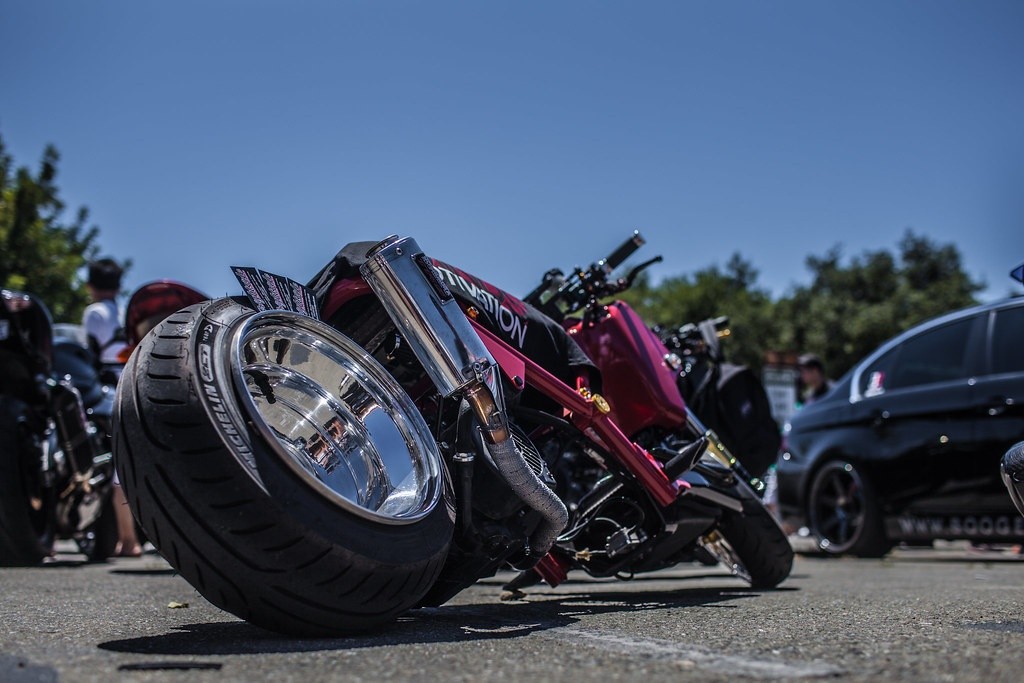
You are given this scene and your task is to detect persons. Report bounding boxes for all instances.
[795,352,830,404]
[83,259,157,556]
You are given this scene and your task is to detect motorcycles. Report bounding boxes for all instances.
[0,231,796,636]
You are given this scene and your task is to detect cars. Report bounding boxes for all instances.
[774,294,1024,559]
